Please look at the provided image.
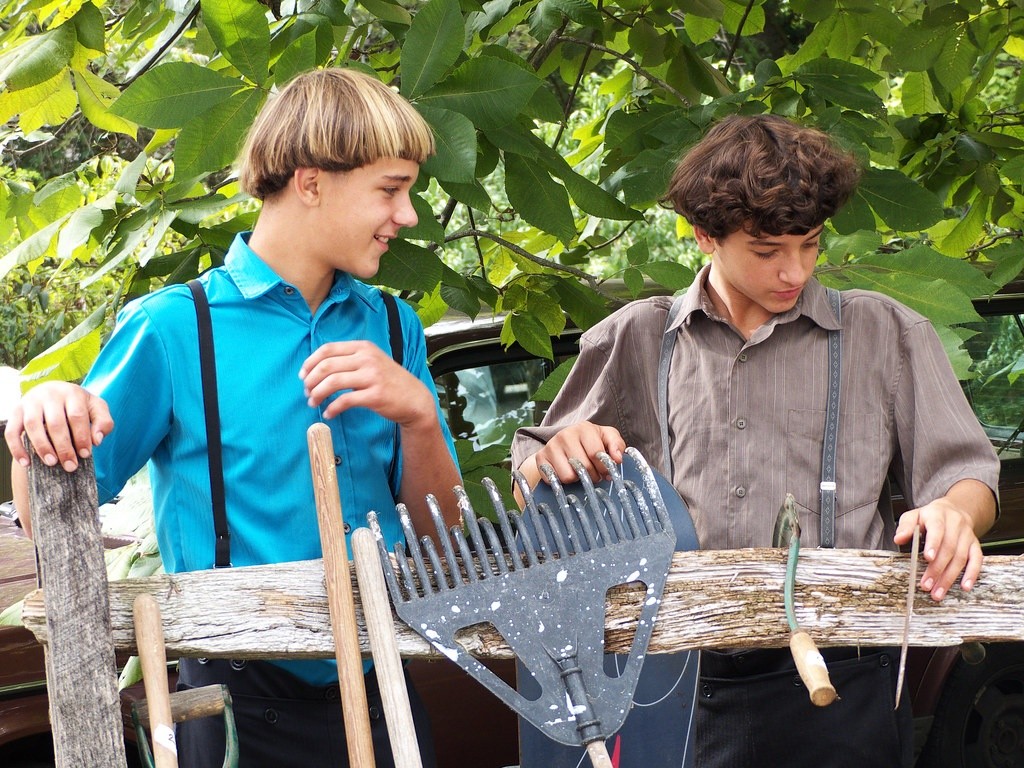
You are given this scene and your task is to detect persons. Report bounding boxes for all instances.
[509,114,1004,768]
[6,65,471,768]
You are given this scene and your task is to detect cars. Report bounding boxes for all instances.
[1,281,1023,767]
